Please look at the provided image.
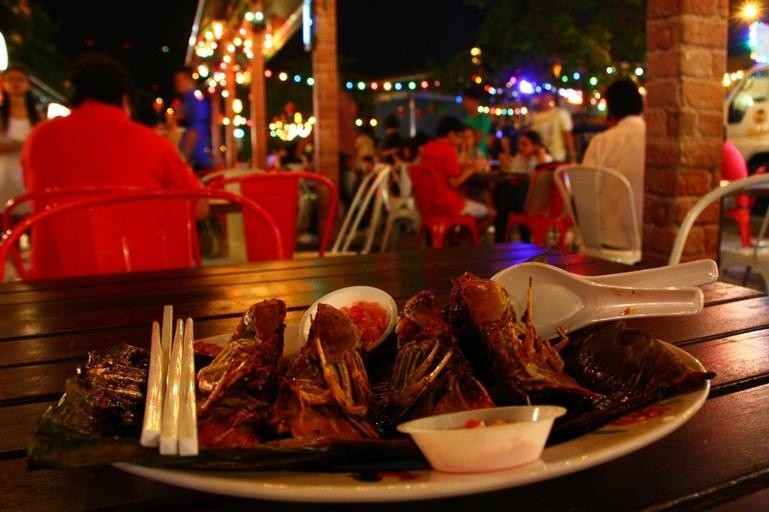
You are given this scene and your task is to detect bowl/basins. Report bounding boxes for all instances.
[298,285,398,361]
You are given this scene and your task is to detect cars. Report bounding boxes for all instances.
[722,61,769,218]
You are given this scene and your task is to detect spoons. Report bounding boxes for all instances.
[474,262,725,347]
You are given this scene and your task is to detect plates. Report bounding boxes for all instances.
[56,335,711,503]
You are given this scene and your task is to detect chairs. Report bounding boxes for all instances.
[196,156,337,261]
[668,173,769,294]
[293,156,644,265]
[1,186,286,282]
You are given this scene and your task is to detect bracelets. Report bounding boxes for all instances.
[568,152,576,159]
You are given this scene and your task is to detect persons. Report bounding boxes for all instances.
[145,106,191,169]
[0,62,47,226]
[17,48,211,225]
[169,63,218,170]
[527,81,581,166]
[581,76,650,243]
[337,90,553,248]
[266,134,316,230]
[716,124,751,213]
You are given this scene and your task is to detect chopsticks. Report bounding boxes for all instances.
[138,302,200,457]
